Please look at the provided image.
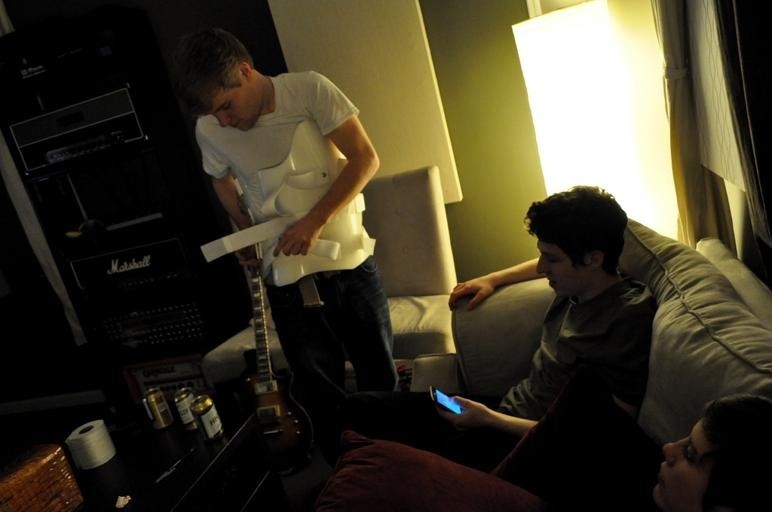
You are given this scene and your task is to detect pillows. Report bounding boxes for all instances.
[491,366,661,510]
[316,430,562,508]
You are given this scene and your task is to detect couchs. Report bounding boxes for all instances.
[452,217,771,444]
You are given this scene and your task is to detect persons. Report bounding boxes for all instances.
[652,391,772,511]
[319,186,657,470]
[165,27,400,470]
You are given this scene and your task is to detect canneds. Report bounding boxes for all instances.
[190,394,224,441]
[141,387,174,430]
[174,387,200,432]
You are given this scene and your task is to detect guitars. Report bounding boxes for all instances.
[238,245,315,474]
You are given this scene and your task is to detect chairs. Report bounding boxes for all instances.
[201,166,454,397]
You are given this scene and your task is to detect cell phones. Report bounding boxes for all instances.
[429,385,462,415]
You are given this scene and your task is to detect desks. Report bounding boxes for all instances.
[65,398,276,512]
